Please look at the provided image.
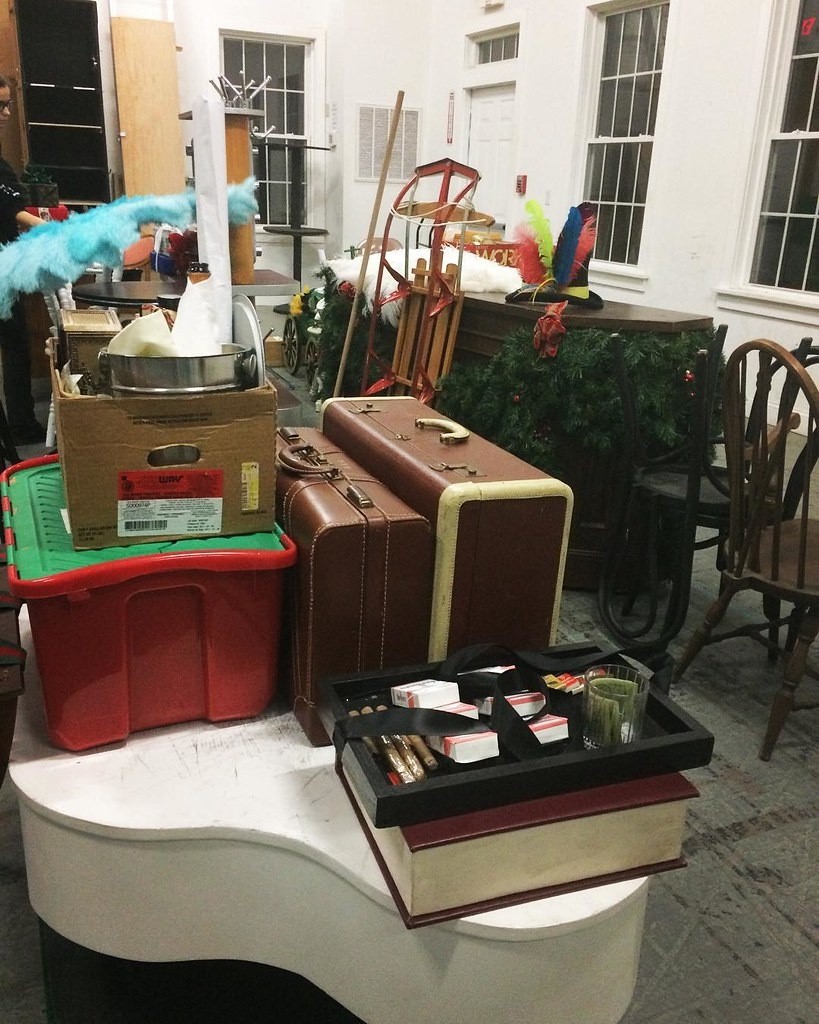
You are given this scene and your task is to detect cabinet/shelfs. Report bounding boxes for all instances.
[8,0,114,210]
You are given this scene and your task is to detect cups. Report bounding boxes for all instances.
[583,664,650,751]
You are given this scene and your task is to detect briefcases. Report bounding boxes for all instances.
[320,394,574,664]
[268,425,437,747]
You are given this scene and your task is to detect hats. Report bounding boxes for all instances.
[504,199,606,311]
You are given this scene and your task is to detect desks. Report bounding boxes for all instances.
[6,595,648,1024]
[449,293,715,381]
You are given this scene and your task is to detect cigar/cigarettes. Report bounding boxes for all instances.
[349,705,438,785]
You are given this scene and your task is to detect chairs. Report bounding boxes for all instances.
[601,323,819,761]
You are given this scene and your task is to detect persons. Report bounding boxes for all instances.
[0,75,58,448]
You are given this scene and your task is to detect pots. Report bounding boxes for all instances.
[98,343,258,396]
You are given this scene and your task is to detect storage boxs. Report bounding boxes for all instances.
[1,338,713,930]
[445,240,523,270]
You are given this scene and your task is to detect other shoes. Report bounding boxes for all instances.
[8,419,47,446]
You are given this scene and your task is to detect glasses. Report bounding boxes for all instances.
[0,100,15,113]
